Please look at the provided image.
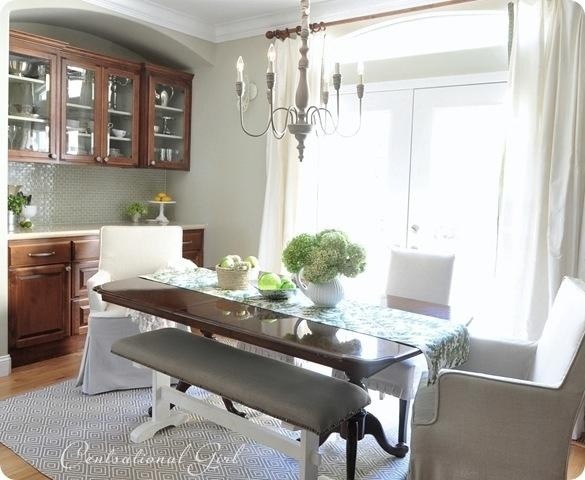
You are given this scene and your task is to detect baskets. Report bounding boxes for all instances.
[216,255,251,290]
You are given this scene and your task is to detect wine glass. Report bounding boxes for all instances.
[9,125,17,150]
[22,205,37,227]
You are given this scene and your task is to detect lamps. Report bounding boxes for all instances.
[236,1,364,161]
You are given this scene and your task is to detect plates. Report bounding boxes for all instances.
[250,279,300,301]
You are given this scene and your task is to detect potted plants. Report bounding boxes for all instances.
[9,192,37,228]
[126,202,149,223]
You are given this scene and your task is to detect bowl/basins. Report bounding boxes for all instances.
[113,129,127,137]
[9,59,33,77]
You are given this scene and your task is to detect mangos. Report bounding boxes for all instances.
[153,192,171,202]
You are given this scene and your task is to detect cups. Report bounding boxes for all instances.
[154,148,182,161]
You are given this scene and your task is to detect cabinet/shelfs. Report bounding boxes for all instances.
[8,28,194,172]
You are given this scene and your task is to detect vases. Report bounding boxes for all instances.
[296,265,344,308]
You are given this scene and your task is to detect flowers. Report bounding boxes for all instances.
[282,230,367,284]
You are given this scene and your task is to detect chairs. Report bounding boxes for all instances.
[332,251,458,443]
[403,275,585,480]
[75,226,192,396]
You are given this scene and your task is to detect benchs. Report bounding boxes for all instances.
[111,327,372,479]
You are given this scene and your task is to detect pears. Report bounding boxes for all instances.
[219,256,230,265]
[259,272,280,290]
[221,260,234,268]
[280,278,295,290]
[247,255,257,268]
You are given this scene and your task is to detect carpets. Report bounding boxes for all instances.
[2,338,411,479]
